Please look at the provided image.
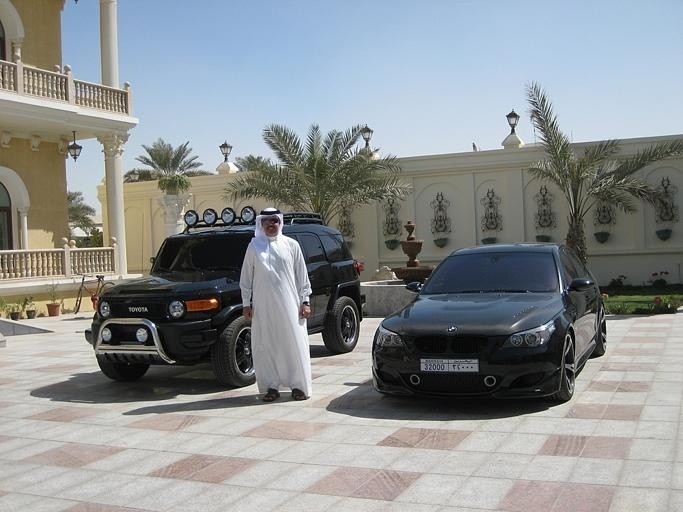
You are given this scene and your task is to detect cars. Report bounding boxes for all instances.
[369,240,609,405]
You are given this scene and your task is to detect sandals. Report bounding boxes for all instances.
[263,388,280,402]
[292,389,306,400]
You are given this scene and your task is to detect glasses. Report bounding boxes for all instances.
[262,218,278,224]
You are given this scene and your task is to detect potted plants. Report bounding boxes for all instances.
[23,296,37,319]
[0,295,25,321]
[46,283,62,316]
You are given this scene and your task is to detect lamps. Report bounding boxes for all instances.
[359,124,373,149]
[68,131,83,162]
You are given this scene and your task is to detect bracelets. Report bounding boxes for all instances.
[302,301,310,306]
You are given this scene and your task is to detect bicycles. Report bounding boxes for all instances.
[73,272,116,315]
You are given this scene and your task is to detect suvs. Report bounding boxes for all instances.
[84,207,370,390]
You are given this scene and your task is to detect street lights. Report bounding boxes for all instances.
[359,124,375,148]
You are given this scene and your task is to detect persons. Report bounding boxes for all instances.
[237,207,312,401]
